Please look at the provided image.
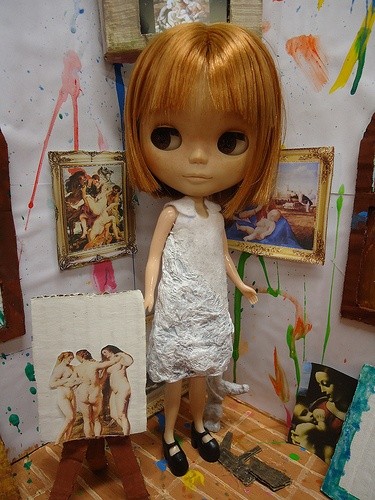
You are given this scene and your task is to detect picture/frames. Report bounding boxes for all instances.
[49,148,138,270]
[97,0,262,65]
[227,146,337,266]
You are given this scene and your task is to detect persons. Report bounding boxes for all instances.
[123,20,290,476]
[294,402,326,455]
[291,366,350,458]
